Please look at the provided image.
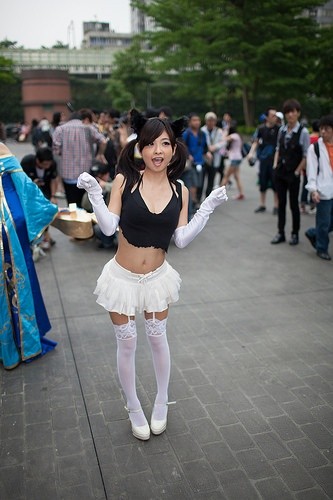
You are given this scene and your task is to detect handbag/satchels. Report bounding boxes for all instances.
[241,142,250,157]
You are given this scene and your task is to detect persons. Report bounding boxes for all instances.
[305,115,333,261]
[0,125,69,371]
[4,108,283,249]
[270,99,310,245]
[76,116,227,441]
[299,119,320,216]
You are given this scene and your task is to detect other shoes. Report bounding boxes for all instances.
[256,206,265,212]
[270,233,285,244]
[316,249,331,260]
[289,234,299,246]
[273,208,278,215]
[235,195,244,200]
[305,228,317,248]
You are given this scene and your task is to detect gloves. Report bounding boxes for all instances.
[76,171,119,236]
[174,185,229,250]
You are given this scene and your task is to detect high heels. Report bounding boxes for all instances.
[151,396,170,434]
[124,406,151,441]
